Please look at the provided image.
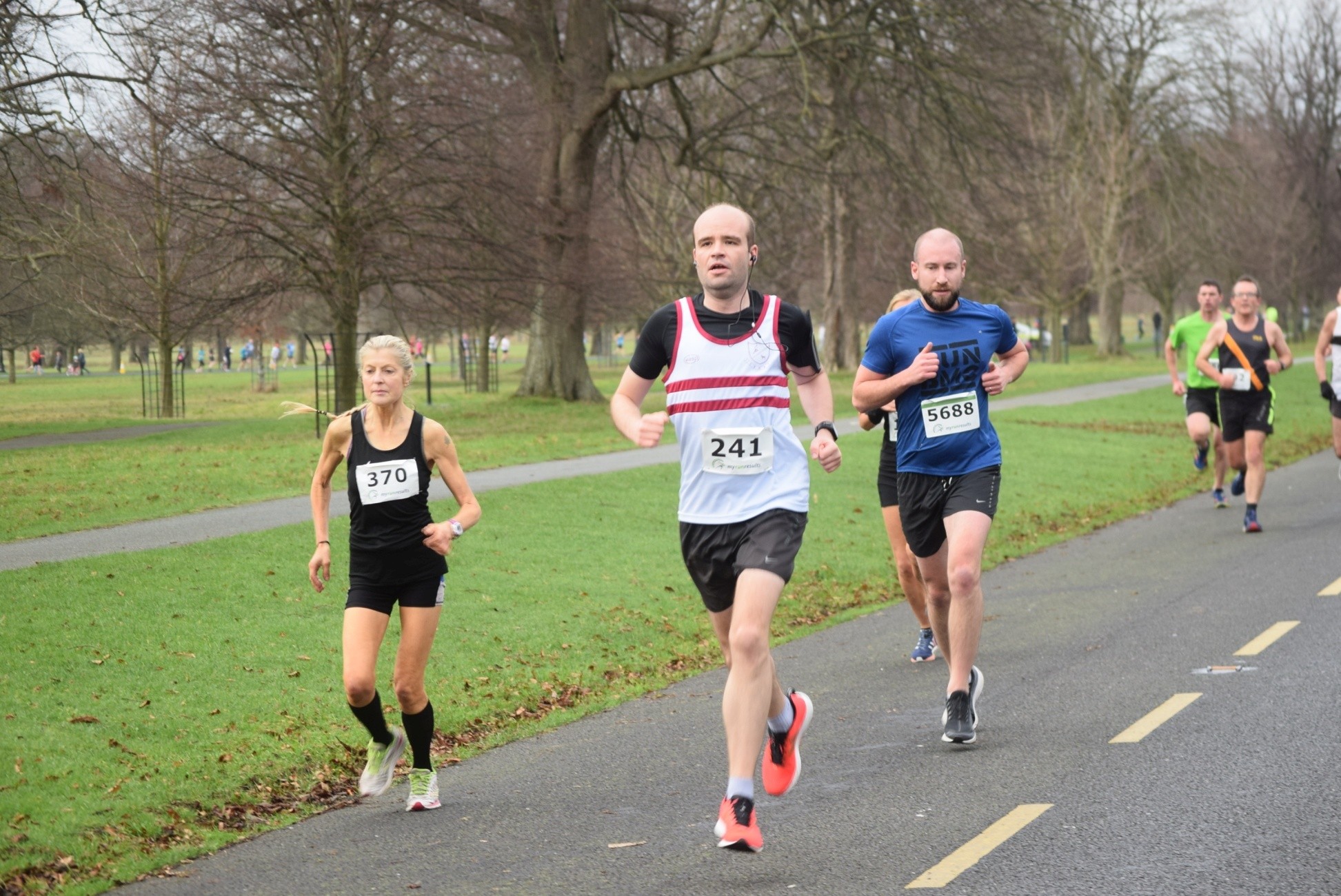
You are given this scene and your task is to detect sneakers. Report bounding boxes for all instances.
[405,768,442,812]
[940,690,976,744]
[358,723,406,799]
[1192,438,1210,472]
[941,664,984,729]
[761,691,814,796]
[713,794,763,853]
[910,628,938,663]
[1230,470,1247,496]
[1211,487,1229,508]
[1243,508,1261,532]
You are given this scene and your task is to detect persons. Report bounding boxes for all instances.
[852,228,1029,748]
[858,288,940,663]
[1194,273,1293,533]
[307,335,481,813]
[28,344,91,377]
[1314,286,1341,459]
[1138,313,1145,340]
[175,335,510,376]
[609,200,841,855]
[1164,280,1235,509]
[616,331,625,356]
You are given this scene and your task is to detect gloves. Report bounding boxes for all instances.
[1320,380,1334,400]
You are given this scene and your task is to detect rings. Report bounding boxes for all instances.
[989,387,993,392]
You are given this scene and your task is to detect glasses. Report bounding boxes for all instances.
[1234,292,1257,298]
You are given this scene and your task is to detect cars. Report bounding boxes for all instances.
[1013,321,1052,347]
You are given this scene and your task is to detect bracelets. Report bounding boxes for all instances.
[317,541,331,546]
[1279,361,1285,370]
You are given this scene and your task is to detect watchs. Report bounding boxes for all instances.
[814,420,838,441]
[449,518,463,538]
